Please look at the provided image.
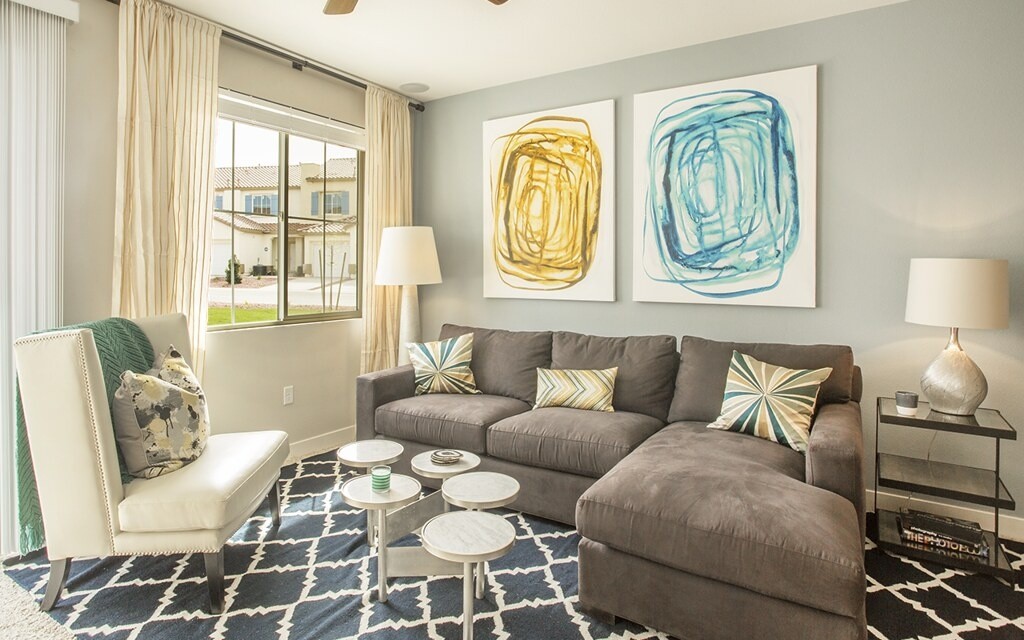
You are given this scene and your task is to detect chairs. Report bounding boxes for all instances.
[11,311,292,617]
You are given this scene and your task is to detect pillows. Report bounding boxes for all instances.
[707,350,834,456]
[532,366,618,413]
[109,343,212,481]
[407,332,483,397]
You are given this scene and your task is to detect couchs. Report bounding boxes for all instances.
[356,321,678,525]
[569,334,868,640]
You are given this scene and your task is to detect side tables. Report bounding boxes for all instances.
[874,396,1018,581]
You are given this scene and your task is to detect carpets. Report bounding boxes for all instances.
[3,446,1024,640]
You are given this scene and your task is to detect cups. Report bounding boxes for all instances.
[370,464,391,493]
[895,390,919,416]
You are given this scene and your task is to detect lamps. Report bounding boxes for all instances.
[905,255,1009,417]
[373,226,444,367]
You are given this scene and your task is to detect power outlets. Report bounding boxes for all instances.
[284,386,294,406]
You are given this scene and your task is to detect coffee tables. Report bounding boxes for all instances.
[334,438,522,640]
